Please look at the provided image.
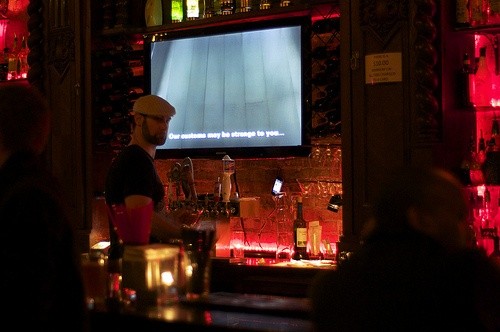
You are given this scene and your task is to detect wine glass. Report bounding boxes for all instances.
[308,137,342,166]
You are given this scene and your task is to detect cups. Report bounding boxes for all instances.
[80,254,108,306]
[174,217,343,262]
[297,176,342,197]
[179,244,210,298]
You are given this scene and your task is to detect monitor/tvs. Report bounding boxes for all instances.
[144,13,312,158]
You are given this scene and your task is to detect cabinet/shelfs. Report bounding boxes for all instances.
[309,0,342,135]
[441,0,500,187]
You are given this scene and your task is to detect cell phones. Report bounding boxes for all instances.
[272,175,284,196]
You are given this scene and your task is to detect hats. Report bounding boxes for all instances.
[132,94,176,117]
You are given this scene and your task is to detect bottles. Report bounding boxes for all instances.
[90,0,144,147]
[292,203,309,253]
[144,0,291,28]
[446,1,499,245]
[310,15,342,143]
[0,35,28,81]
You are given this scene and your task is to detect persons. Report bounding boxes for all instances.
[105,95,175,273]
[0,85,85,332]
[312,167,500,332]
